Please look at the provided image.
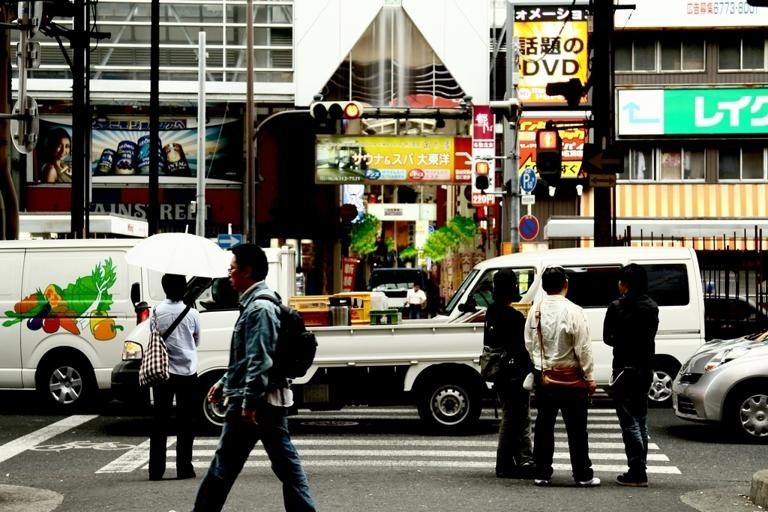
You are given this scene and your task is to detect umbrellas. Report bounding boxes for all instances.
[126,223,235,280]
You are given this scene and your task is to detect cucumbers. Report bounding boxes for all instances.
[2,318,23,327]
[4,304,42,317]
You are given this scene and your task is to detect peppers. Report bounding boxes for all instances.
[90,310,108,334]
[93,318,124,340]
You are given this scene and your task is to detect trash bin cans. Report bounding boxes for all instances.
[327,295,352,325]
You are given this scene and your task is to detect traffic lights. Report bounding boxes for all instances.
[537,129,561,181]
[476,161,490,191]
[310,101,362,120]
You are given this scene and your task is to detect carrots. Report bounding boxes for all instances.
[56,312,80,335]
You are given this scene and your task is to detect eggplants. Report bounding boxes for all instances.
[27,302,51,330]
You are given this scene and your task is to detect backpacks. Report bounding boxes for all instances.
[235,291,316,381]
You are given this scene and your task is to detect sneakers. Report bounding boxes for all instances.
[534,477,553,488]
[146,471,165,481]
[176,472,197,480]
[495,467,526,480]
[574,476,594,487]
[623,470,646,477]
[617,476,648,487]
[517,454,536,466]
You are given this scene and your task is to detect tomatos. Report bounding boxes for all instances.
[42,313,60,333]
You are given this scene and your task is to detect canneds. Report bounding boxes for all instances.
[96,135,191,175]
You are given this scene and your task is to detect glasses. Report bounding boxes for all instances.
[227,266,244,272]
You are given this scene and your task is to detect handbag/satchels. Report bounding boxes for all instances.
[537,367,595,393]
[137,333,170,388]
[478,353,507,383]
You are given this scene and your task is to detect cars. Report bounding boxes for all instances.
[701,291,767,340]
[669,332,768,444]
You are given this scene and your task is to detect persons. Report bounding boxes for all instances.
[483,267,538,480]
[603,263,659,487]
[423,278,440,318]
[148,273,201,481]
[524,267,602,487]
[404,284,427,319]
[39,127,72,183]
[169,242,318,511]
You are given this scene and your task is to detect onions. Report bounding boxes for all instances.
[30,294,37,307]
[14,296,32,313]
